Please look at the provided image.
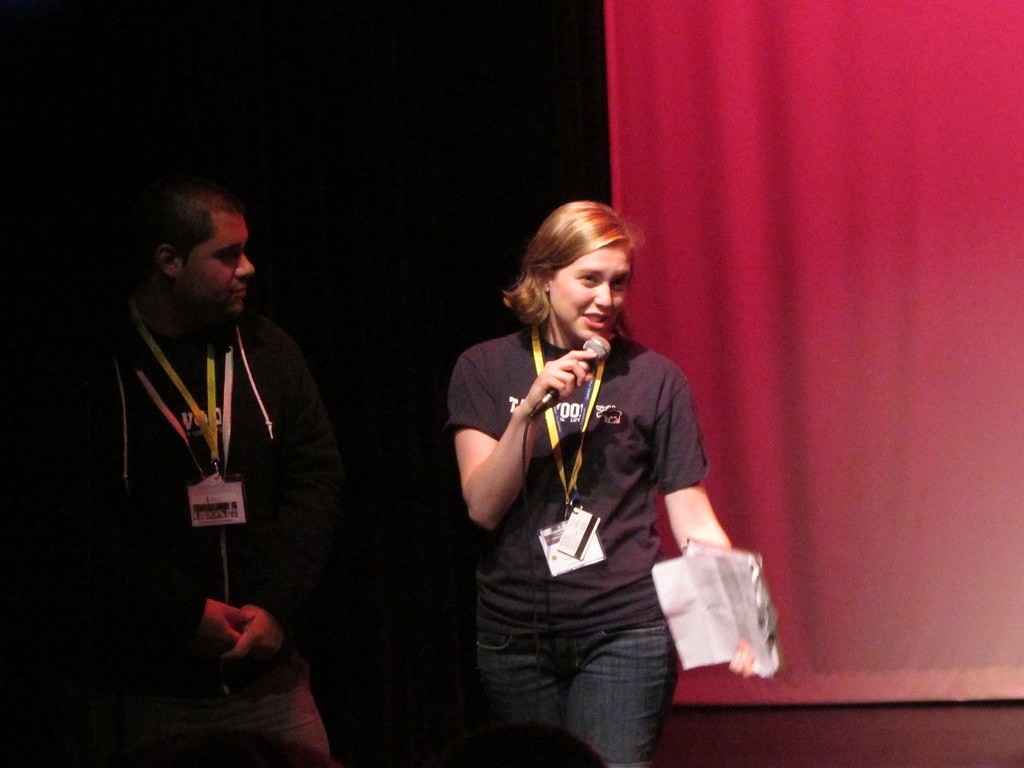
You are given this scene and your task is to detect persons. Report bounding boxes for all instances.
[91,721,606,766]
[55,184,343,768]
[445,200,767,767]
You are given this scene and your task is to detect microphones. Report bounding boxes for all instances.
[529,335,611,420]
